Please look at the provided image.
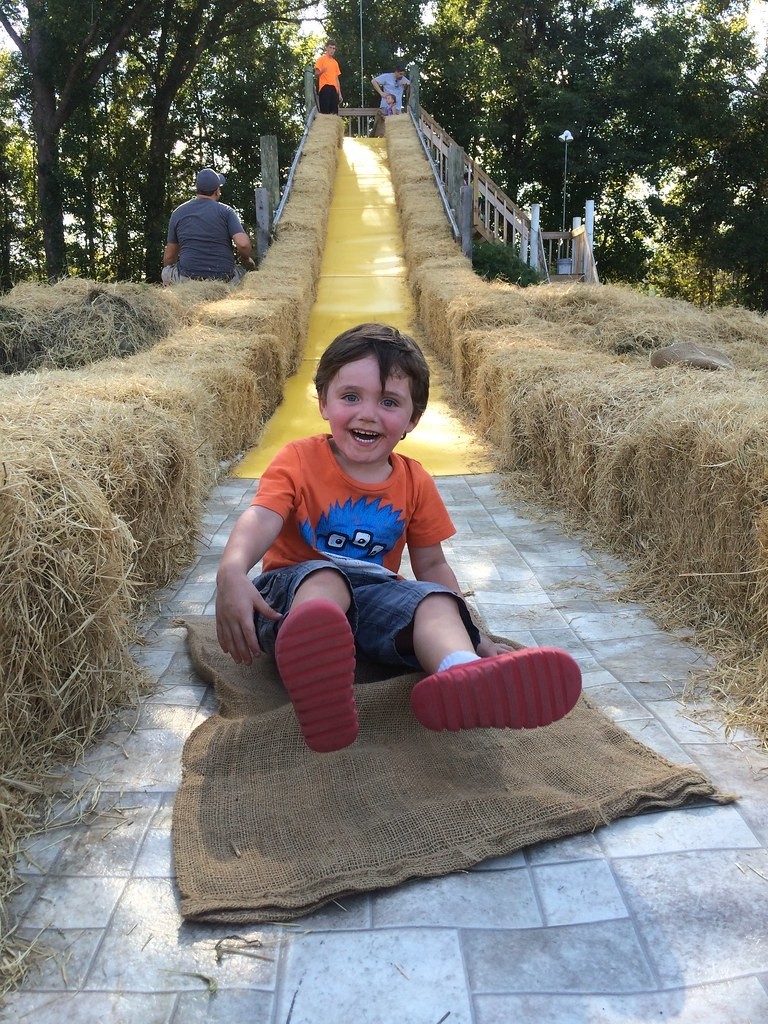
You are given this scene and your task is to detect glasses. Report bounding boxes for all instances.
[395,79,398,87]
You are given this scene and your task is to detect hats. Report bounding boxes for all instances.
[197,169,227,191]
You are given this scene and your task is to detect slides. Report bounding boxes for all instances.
[229,133,494,479]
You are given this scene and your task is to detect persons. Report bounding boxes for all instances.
[217,322,582,753]
[315,40,343,116]
[371,66,410,116]
[161,169,251,285]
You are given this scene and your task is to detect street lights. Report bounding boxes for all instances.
[557,128,574,276]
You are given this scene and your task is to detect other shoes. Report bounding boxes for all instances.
[274,597,359,752]
[411,646,584,732]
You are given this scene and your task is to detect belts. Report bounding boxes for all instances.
[190,273,235,283]
